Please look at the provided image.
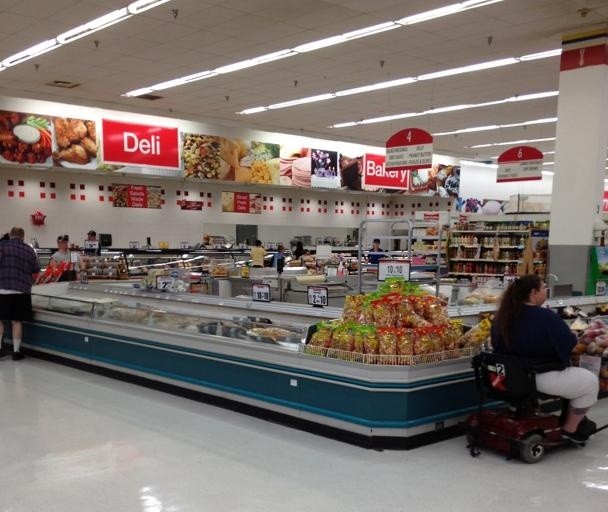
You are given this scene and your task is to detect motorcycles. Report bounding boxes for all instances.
[464,352,602,464]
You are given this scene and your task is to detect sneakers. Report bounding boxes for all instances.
[561,428,589,443]
[12,351,25,360]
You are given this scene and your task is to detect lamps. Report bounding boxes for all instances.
[234,47,563,116]
[540,162,555,165]
[326,89,560,129]
[1,1,172,73]
[466,135,557,151]
[121,1,504,99]
[431,116,559,137]
[490,151,555,160]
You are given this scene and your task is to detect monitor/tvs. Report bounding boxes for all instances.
[100,233,112,246]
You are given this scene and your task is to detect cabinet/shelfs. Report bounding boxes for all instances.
[447,228,549,289]
[358,218,441,297]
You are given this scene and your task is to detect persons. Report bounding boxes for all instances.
[362,238,386,265]
[249,239,305,268]
[0,224,105,362]
[490,275,600,446]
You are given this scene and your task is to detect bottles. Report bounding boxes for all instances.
[451,247,522,261]
[453,220,549,232]
[449,232,529,248]
[450,260,518,277]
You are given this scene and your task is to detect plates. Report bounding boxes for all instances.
[0,121,53,168]
[53,130,97,170]
